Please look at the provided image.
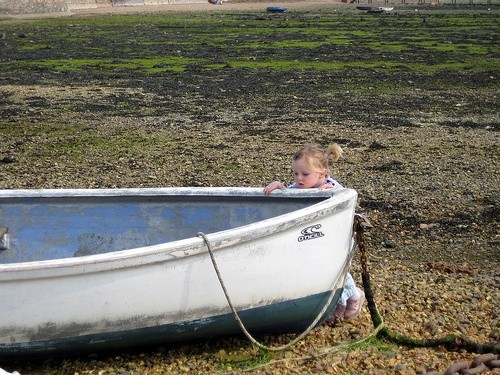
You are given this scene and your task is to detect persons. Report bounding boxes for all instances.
[263,138,367,323]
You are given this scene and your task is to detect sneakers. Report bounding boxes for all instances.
[343,287,365,320]
[329,304,347,321]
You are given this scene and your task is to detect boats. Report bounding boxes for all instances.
[0,185,358,364]
